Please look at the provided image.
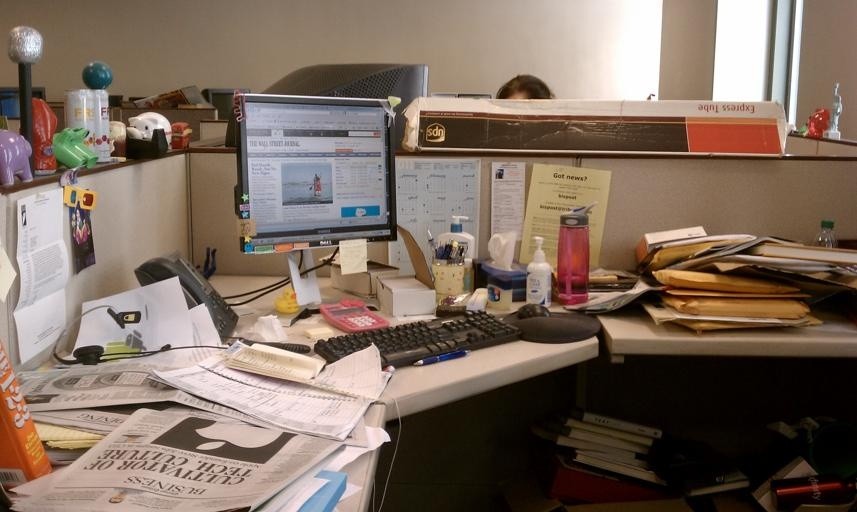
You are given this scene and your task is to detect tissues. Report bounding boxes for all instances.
[472,231,527,301]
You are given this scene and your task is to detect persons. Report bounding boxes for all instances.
[491,71,556,99]
[313,173,324,197]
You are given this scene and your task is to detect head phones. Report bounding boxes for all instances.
[52,305,126,365]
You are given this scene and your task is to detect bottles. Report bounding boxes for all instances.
[811,219,838,249]
[556,201,594,306]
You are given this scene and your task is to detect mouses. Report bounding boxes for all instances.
[517,304,552,320]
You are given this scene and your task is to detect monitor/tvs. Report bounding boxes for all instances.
[0,87,46,120]
[201,88,250,120]
[232,93,398,328]
[224,63,428,147]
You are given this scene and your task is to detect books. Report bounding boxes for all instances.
[557,396,669,485]
[628,233,857,333]
[685,464,755,498]
[0,333,54,489]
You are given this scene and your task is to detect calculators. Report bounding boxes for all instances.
[319,298,390,333]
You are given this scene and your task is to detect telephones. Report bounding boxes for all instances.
[134,249,239,345]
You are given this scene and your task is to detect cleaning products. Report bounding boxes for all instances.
[436,215,475,260]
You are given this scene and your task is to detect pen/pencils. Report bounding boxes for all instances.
[413,349,470,365]
[426,229,465,265]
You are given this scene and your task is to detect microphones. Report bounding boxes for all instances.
[99,344,171,363]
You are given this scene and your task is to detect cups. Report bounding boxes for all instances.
[433,262,465,304]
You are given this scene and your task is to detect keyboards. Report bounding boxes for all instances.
[313,307,521,370]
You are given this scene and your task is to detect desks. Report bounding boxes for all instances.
[0,145,386,512]
[189,146,600,511]
[579,148,856,512]
